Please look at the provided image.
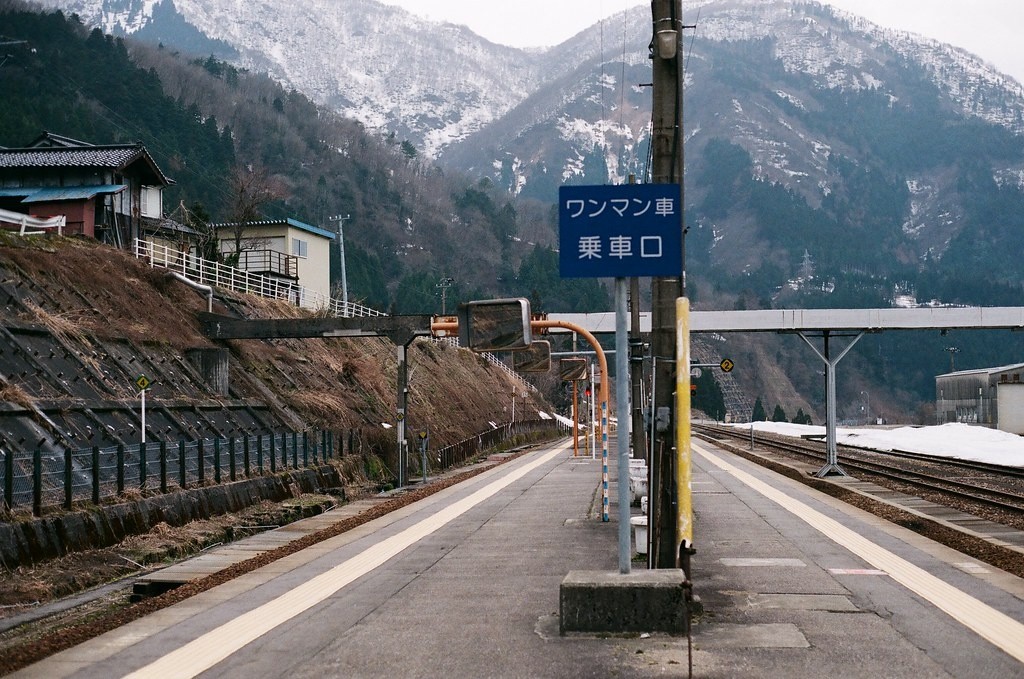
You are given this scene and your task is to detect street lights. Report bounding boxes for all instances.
[861,391,869,425]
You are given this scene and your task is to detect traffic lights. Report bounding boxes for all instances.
[586,386,592,396]
